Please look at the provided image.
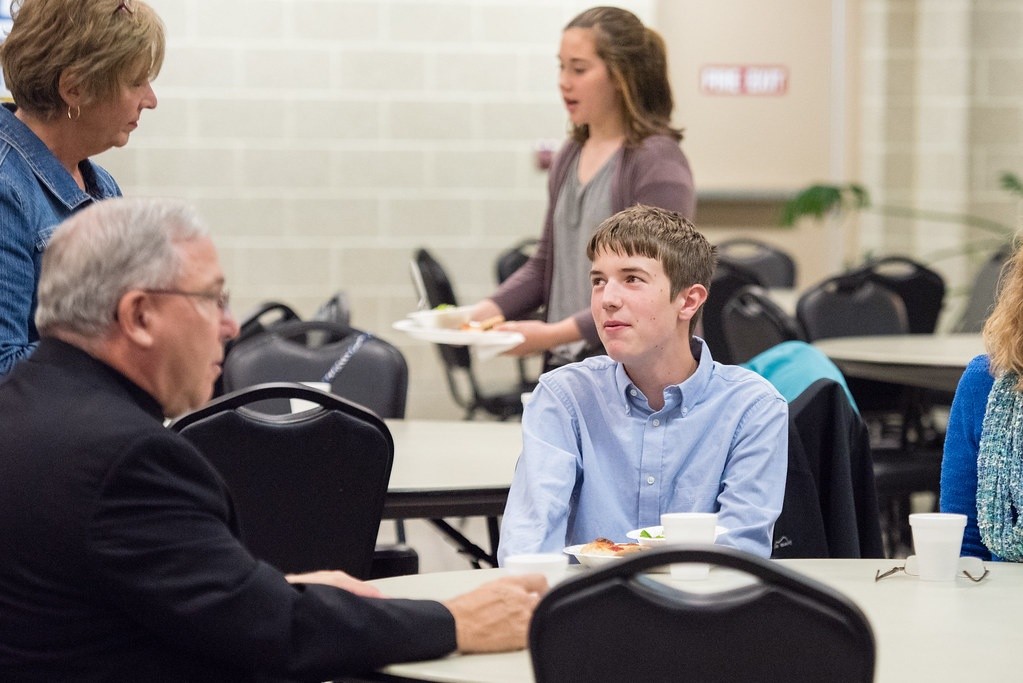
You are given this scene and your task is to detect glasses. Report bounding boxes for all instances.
[876,553,988,583]
[113,0,136,17]
[112,280,231,328]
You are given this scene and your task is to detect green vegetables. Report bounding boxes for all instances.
[640,529,664,538]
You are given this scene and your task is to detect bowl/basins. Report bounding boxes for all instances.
[406,307,478,329]
[562,543,634,569]
[625,525,726,545]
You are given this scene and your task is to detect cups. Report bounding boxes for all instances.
[661,512,718,580]
[504,552,568,589]
[288,381,331,414]
[909,512,967,582]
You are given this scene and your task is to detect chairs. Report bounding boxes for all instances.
[168,238,947,682]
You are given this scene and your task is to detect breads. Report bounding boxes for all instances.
[579,538,642,555]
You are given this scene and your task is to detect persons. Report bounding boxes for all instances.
[445,5,695,371]
[497,206,789,566]
[0,198,549,683]
[0,0,165,378]
[939,227,1023,562]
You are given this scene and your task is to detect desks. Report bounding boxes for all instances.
[364,560,1023,682]
[381,419,524,567]
[812,335,985,393]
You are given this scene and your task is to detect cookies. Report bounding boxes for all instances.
[476,316,505,330]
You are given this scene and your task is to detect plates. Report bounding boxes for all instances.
[390,321,524,347]
